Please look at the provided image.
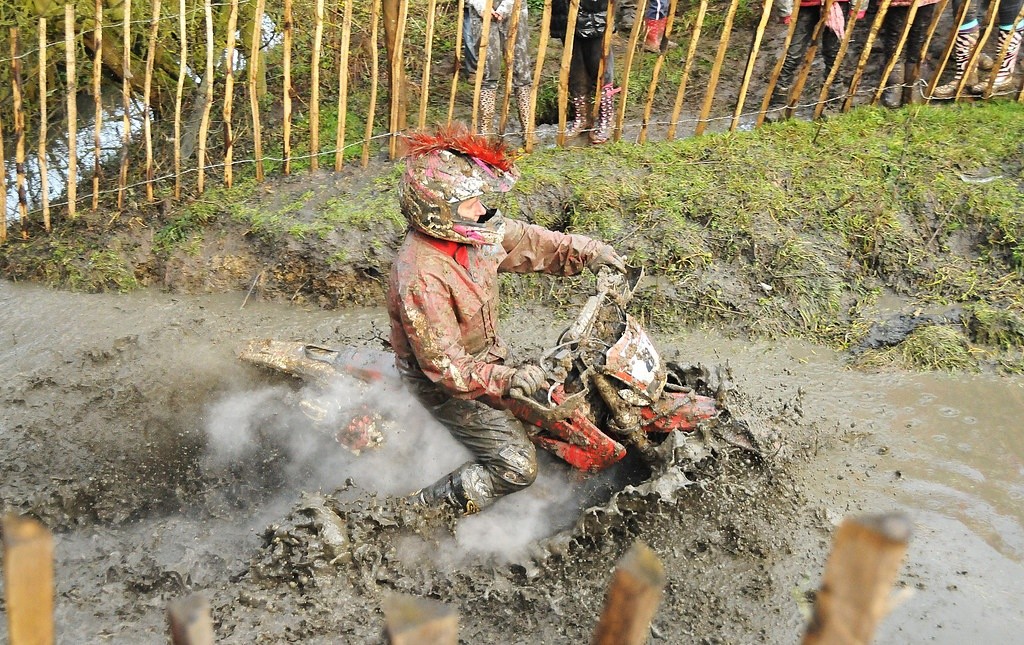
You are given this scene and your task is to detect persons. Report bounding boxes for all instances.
[399,146,628,521]
[463,0,1024,145]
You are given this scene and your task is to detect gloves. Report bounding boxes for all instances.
[587,245,628,278]
[509,364,547,397]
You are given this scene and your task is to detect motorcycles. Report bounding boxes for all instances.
[235,256,775,499]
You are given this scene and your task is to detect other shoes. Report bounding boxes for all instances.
[765,105,786,124]
[821,101,844,118]
[977,51,990,68]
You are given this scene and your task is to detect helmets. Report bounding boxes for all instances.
[397,123,517,248]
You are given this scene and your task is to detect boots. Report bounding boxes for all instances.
[590,81,621,144]
[400,465,482,522]
[478,89,496,136]
[641,17,680,56]
[970,19,1024,95]
[932,19,981,98]
[514,86,533,146]
[567,94,591,135]
[881,63,924,109]
